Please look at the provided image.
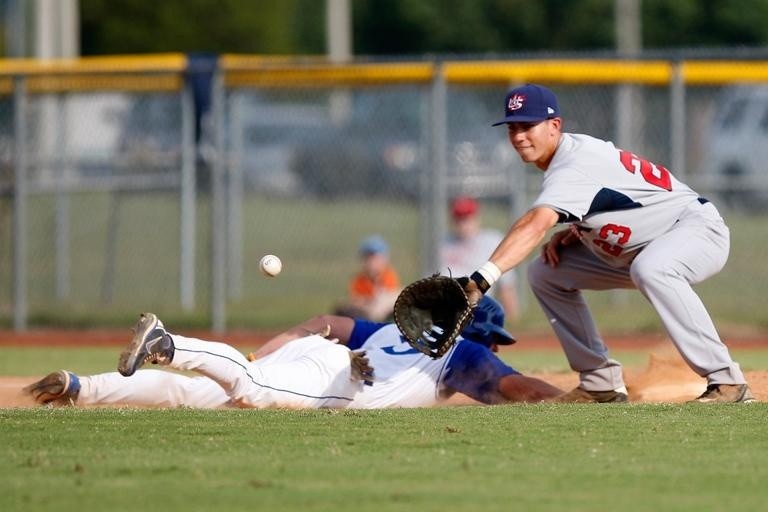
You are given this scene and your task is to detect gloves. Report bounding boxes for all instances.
[348,350,374,383]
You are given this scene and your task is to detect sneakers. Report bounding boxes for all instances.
[686,385,756,405]
[550,387,628,404]
[22,369,82,405]
[117,312,168,377]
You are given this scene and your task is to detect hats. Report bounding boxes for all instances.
[452,200,478,221]
[492,85,561,127]
[360,239,386,257]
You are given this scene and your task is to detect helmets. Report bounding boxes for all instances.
[462,295,516,346]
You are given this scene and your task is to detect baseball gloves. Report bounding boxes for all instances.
[394,271,491,359]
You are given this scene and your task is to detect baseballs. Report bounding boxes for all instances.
[259,254,281,278]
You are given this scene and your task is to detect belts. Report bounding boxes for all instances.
[363,366,374,386]
[697,197,709,205]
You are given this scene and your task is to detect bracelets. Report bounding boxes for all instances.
[470,263,502,294]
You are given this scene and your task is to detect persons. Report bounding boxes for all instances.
[16,296,566,409]
[393,82,752,412]
[436,200,523,320]
[335,236,404,323]
